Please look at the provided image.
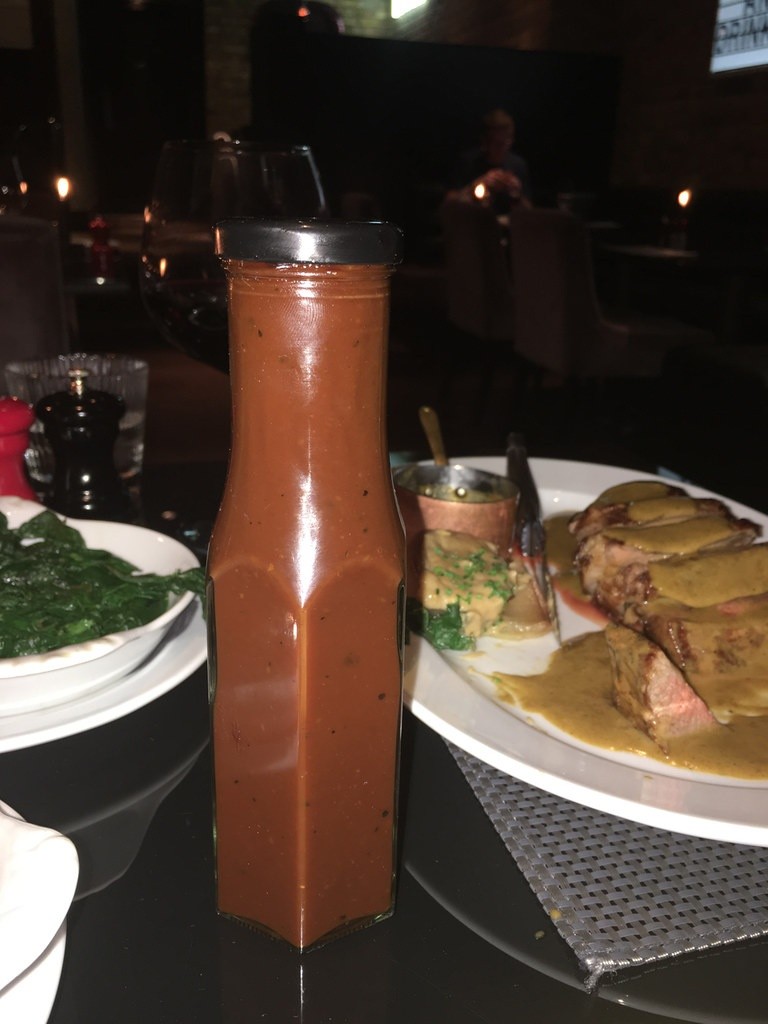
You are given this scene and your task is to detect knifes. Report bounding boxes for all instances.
[503,432,565,644]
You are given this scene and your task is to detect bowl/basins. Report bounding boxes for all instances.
[0,516,199,716]
[0,801,67,1024]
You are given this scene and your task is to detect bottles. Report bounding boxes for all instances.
[37,369,129,523]
[0,395,40,504]
[201,142,407,954]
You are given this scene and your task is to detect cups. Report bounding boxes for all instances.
[5,355,148,484]
[395,467,522,553]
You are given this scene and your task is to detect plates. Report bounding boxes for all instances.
[0,594,208,750]
[393,456,768,849]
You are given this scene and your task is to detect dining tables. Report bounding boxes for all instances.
[47,459,686,1023]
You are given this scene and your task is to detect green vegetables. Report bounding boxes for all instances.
[1,507,208,663]
[403,543,517,651]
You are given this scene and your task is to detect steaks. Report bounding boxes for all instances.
[407,528,509,628]
[566,480,768,747]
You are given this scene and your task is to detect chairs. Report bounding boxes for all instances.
[340,192,440,301]
[437,201,545,417]
[511,207,692,454]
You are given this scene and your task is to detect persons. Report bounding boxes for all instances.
[435,109,532,211]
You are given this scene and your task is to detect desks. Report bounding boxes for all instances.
[594,243,699,305]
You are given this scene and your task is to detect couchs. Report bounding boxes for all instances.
[604,187,768,434]
